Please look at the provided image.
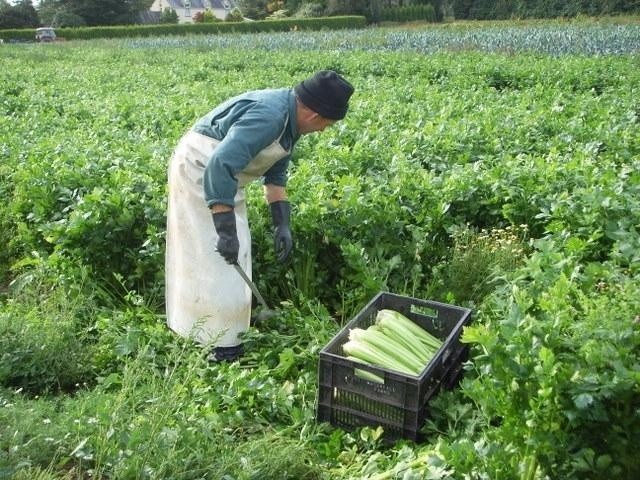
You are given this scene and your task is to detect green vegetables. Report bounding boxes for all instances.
[328,308,446,428]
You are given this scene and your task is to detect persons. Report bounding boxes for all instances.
[164,70,356,367]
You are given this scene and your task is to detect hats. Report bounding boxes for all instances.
[295,72,356,121]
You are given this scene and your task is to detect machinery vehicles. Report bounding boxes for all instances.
[9,27,63,42]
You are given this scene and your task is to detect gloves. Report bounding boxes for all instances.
[211,207,239,267]
[270,202,293,264]
